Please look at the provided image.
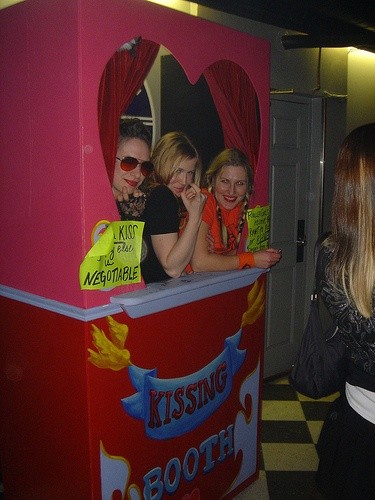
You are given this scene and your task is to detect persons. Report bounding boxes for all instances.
[315,122,375,500]
[139,131,207,284]
[111,118,154,218]
[179,147,280,275]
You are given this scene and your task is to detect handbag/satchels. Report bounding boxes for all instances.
[289,246,347,400]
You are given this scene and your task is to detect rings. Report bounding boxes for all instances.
[128,194,132,199]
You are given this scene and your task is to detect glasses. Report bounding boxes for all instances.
[115,156,154,177]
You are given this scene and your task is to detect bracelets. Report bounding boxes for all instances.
[237,252,254,271]
